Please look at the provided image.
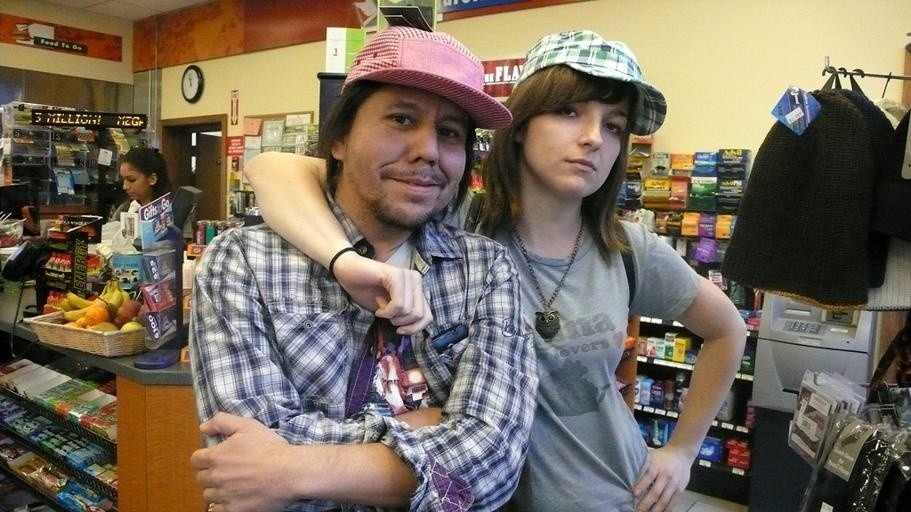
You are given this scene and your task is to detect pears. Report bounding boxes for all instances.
[106,302,119,321]
[92,322,119,332]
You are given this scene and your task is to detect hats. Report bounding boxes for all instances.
[341,25,513,131]
[511,29,667,136]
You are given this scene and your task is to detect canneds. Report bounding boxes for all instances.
[196,220,236,245]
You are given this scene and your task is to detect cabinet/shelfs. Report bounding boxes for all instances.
[2,322,122,511]
[615,147,763,507]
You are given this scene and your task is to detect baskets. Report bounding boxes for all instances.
[22,311,157,358]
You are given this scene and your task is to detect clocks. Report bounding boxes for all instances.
[180,63,206,104]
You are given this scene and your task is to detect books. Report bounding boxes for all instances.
[643,147,752,214]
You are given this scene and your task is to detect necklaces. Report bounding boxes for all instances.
[514,219,586,343]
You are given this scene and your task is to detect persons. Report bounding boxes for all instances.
[245,29,748,510]
[109,143,172,235]
[188,28,541,510]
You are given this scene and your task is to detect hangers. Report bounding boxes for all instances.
[815,64,909,130]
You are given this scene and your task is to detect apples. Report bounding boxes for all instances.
[120,322,143,334]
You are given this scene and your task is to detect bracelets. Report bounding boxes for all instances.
[330,244,356,279]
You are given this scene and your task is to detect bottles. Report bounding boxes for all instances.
[43,290,68,316]
[49,253,72,274]
[662,372,690,414]
[195,219,237,247]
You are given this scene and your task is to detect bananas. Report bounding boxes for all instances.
[61,278,130,322]
